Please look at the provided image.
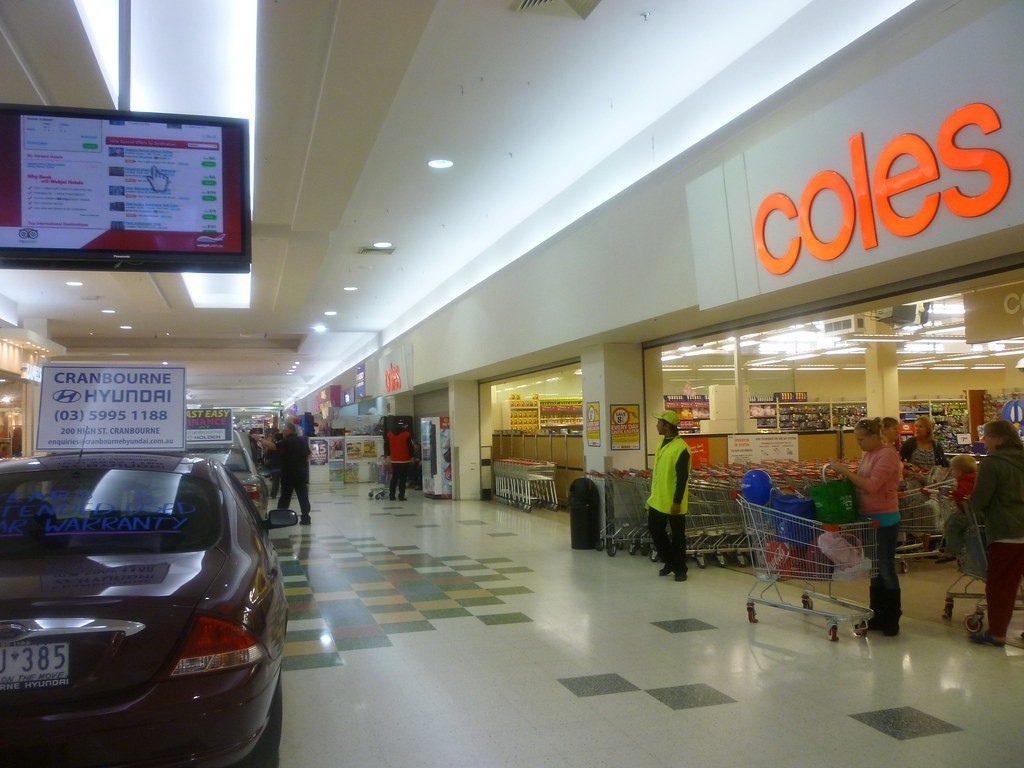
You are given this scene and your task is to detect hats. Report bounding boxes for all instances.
[399,418,408,426]
[654,409,680,426]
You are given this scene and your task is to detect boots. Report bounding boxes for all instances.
[857,587,902,636]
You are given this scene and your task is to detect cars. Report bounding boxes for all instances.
[0,448,303,768]
[188,430,270,520]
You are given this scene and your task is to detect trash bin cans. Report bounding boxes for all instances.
[569,477,600,550]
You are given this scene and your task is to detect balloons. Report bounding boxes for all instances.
[742,469,772,506]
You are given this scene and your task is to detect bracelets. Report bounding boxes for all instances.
[259,438,262,441]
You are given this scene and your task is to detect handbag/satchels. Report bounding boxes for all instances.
[811,464,856,522]
[770,488,811,544]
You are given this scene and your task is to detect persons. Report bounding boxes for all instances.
[646,410,692,582]
[386,422,416,501]
[249,422,312,526]
[935,455,978,563]
[899,414,948,557]
[829,418,901,637]
[971,420,1024,648]
[882,417,924,482]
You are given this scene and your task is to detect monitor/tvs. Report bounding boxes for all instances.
[0,102,252,276]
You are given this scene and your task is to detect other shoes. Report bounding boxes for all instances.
[675,571,687,581]
[935,556,957,564]
[399,497,407,501]
[660,561,673,576]
[300,519,311,524]
[390,496,397,500]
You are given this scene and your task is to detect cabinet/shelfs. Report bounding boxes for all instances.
[897,400,969,440]
[747,402,869,435]
[664,400,711,433]
[507,397,584,434]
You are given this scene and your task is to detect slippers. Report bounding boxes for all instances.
[974,630,1005,649]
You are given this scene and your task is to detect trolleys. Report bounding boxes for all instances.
[368,455,419,500]
[918,486,1024,632]
[731,489,880,641]
[587,456,985,568]
[493,457,560,513]
